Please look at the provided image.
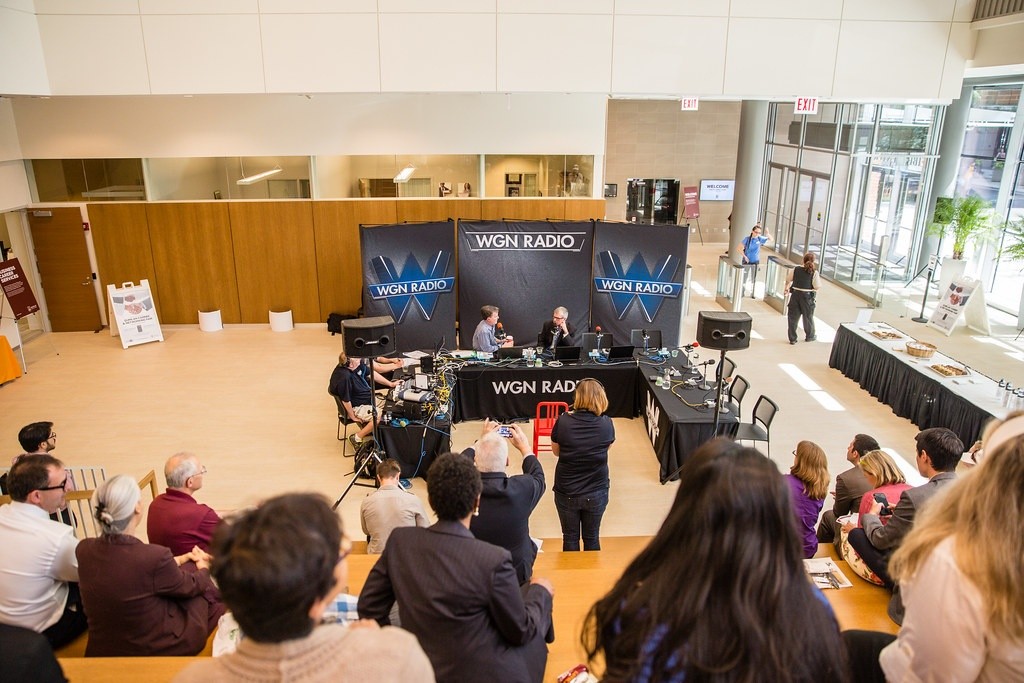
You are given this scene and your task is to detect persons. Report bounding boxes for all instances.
[463,183,471,193]
[842,409,1024,683]
[736,225,775,300]
[460,417,547,589]
[838,448,915,588]
[548,376,615,551]
[1,420,77,531]
[1,453,87,650]
[536,306,578,352]
[328,351,405,454]
[568,164,584,185]
[147,453,233,564]
[725,212,733,253]
[817,433,883,543]
[472,305,514,353]
[859,427,973,594]
[355,453,556,682]
[439,183,450,197]
[627,181,638,212]
[75,473,228,657]
[0,620,73,683]
[175,491,438,682]
[786,250,820,344]
[781,440,831,560]
[583,434,853,683]
[112,293,153,316]
[360,457,431,554]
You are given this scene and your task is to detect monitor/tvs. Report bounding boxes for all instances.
[605,184,617,198]
[700,179,734,201]
[436,336,445,353]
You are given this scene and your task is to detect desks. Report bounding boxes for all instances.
[829,321,1024,453]
[632,347,740,485]
[377,348,453,484]
[450,348,642,423]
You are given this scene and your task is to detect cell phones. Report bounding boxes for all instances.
[873,492,893,515]
[497,425,515,438]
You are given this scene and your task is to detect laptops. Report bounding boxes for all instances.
[553,346,580,363]
[496,346,523,360]
[602,345,635,361]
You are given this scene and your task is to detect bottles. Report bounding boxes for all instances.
[526,347,535,367]
[692,353,700,374]
[662,368,671,390]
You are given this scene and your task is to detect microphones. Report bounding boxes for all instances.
[551,322,558,333]
[676,342,699,348]
[642,329,648,338]
[711,377,734,392]
[497,322,506,337]
[691,359,715,366]
[595,326,603,336]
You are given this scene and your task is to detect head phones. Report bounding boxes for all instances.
[345,358,350,367]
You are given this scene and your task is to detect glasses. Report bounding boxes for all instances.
[792,449,797,456]
[192,465,207,477]
[970,448,984,464]
[36,473,68,492]
[754,231,761,234]
[552,315,563,319]
[49,432,56,439]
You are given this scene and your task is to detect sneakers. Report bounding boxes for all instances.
[348,433,363,453]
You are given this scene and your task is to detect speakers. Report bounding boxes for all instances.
[695,311,752,351]
[341,315,397,358]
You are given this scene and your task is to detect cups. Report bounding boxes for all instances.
[535,359,543,368]
[672,350,678,357]
[655,377,663,386]
[537,347,543,354]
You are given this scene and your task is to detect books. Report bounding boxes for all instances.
[836,512,861,529]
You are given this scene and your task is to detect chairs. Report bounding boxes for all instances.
[532,402,569,459]
[630,328,664,352]
[582,331,615,350]
[720,374,750,422]
[705,356,737,395]
[328,385,375,458]
[734,395,779,459]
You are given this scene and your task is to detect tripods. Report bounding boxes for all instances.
[331,359,408,510]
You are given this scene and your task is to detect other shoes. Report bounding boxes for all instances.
[790,336,799,345]
[805,335,818,342]
